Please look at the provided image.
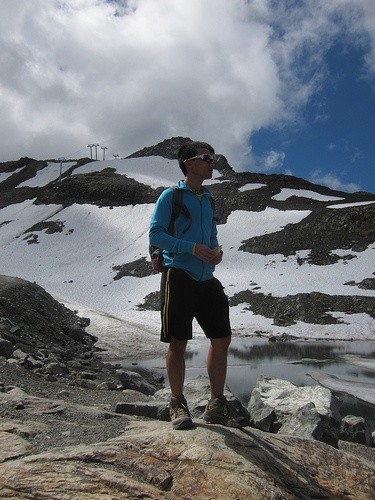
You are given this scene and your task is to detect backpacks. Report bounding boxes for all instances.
[149,186,215,272]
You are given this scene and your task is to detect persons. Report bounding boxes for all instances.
[149,142,251,430]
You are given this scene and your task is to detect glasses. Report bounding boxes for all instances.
[184,154,218,162]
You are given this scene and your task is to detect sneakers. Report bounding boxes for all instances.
[203,395,248,428]
[168,398,194,429]
